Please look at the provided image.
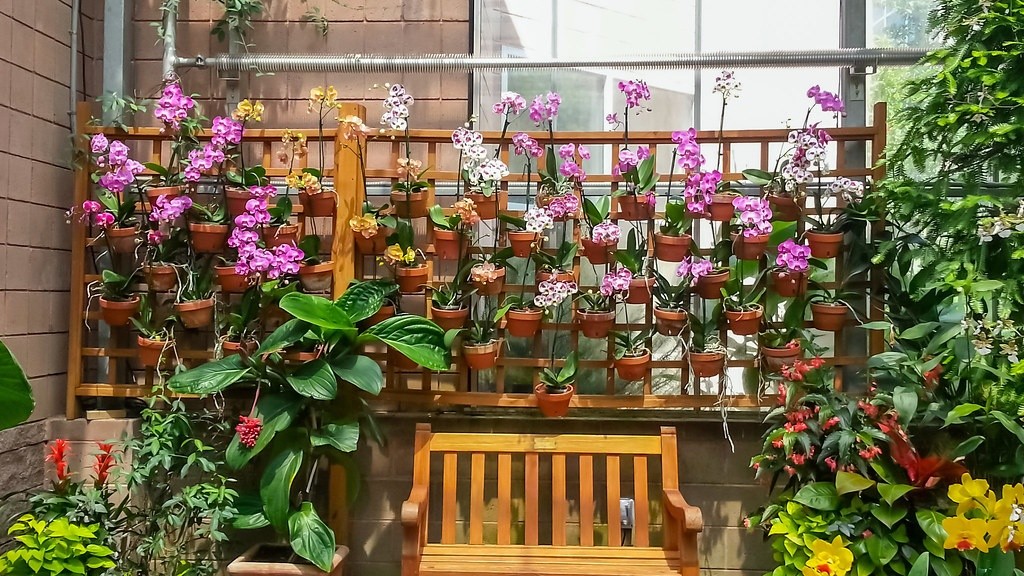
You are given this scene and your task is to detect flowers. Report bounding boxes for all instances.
[744,320,1024,576]
[285,84,342,195]
[230,185,305,286]
[237,418,261,448]
[155,84,193,132]
[147,192,192,245]
[184,117,244,181]
[344,69,1024,310]
[89,132,145,191]
[61,201,115,231]
[230,99,264,122]
[276,127,309,161]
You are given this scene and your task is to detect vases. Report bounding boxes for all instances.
[144,264,177,293]
[535,381,574,418]
[265,221,299,250]
[188,220,228,254]
[213,260,251,293]
[392,190,428,219]
[223,186,266,219]
[100,294,139,328]
[298,191,337,217]
[361,306,397,328]
[353,227,394,256]
[172,297,215,330]
[225,542,350,576]
[393,265,431,292]
[106,221,139,254]
[432,188,847,380]
[146,185,185,209]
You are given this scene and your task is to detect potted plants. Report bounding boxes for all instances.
[128,295,174,370]
[214,291,267,358]
[300,236,335,289]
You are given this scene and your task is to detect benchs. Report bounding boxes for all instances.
[400,422,703,576]
